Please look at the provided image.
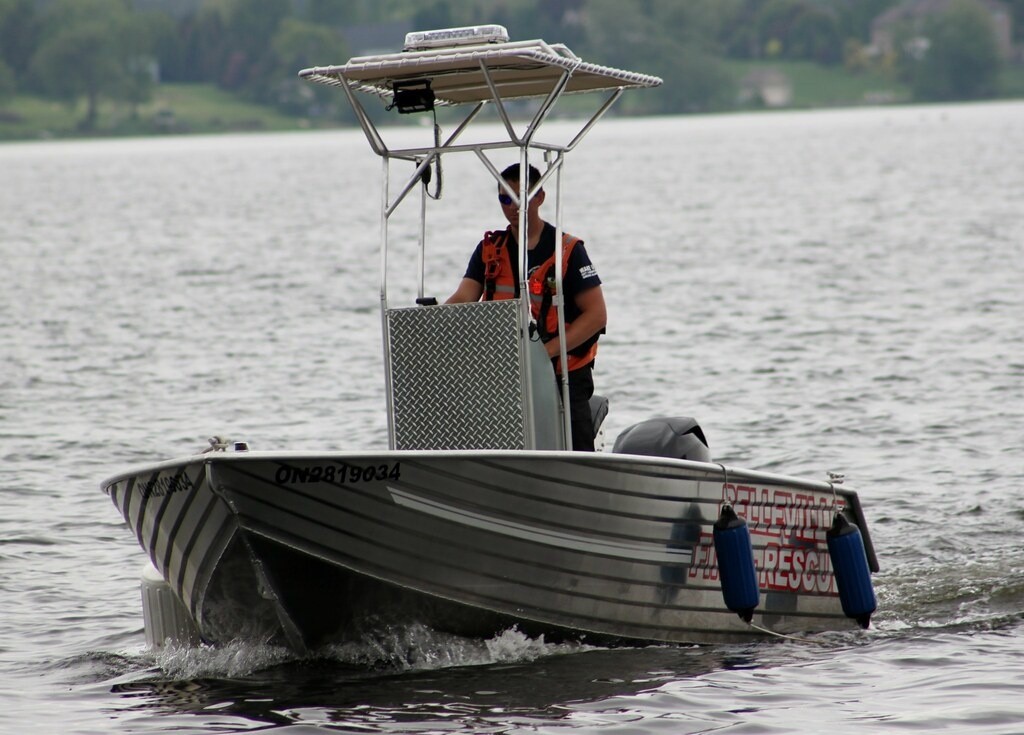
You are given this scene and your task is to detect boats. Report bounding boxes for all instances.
[95,33,882,661]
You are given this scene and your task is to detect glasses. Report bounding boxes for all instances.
[498,193,520,206]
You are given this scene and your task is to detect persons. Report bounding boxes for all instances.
[444,164,607,452]
